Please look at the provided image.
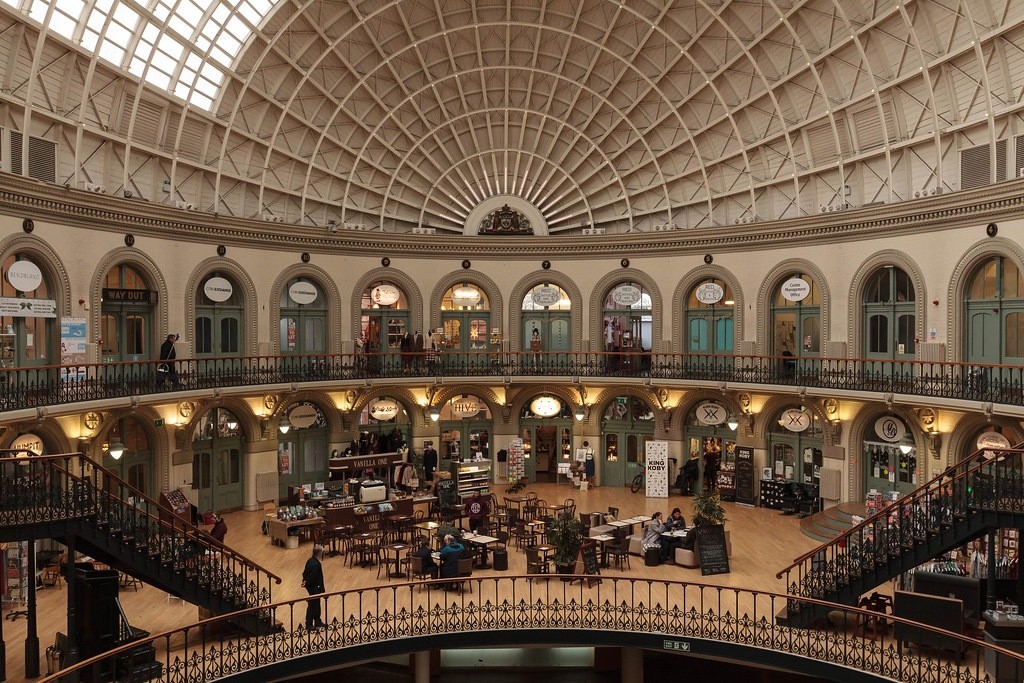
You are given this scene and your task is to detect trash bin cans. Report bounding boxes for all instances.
[776,350,796,380]
[968,365,986,393]
[590,512,603,527]
[286,530,300,549]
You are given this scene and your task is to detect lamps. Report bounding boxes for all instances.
[226,415,237,430]
[899,425,913,453]
[279,409,293,433]
[728,391,738,430]
[108,425,128,460]
[574,406,584,421]
[432,406,439,421]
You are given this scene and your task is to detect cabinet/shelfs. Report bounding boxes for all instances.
[759,480,791,509]
[452,459,491,498]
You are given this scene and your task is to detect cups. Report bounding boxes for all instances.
[670,532,673,536]
[363,480,383,485]
[349,478,358,483]
[474,531,477,537]
[461,530,465,536]
[672,528,675,532]
[436,552,439,557]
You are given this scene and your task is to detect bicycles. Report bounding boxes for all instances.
[631,463,646,493]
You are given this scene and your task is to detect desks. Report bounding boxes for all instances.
[271,496,698,579]
[87,559,109,570]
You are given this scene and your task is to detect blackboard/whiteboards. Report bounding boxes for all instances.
[698,526,730,576]
[431,479,461,516]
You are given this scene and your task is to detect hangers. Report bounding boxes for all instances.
[400,461,414,468]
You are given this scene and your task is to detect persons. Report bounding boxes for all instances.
[421,445,437,492]
[330,448,352,458]
[704,447,719,492]
[437,535,465,588]
[666,508,686,530]
[416,540,439,586]
[437,516,460,548]
[400,331,437,363]
[585,448,595,476]
[156,334,184,391]
[301,545,327,631]
[645,511,670,562]
[464,489,489,534]
[530,328,542,363]
[210,514,228,544]
[397,441,411,463]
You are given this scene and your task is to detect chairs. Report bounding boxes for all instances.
[783,351,796,370]
[264,503,277,535]
[310,492,666,597]
[44,555,67,589]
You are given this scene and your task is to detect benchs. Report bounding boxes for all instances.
[675,531,731,568]
[915,570,983,628]
[893,590,970,667]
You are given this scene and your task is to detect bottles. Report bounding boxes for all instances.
[353,506,371,515]
[344,496,355,507]
[459,480,488,488]
[312,490,322,497]
[379,503,396,512]
[277,506,317,523]
[459,489,488,496]
[413,489,433,499]
[389,490,406,500]
[320,495,333,508]
[329,487,340,499]
[458,457,470,463]
[333,496,343,508]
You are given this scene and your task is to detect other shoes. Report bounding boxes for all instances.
[315,622,328,627]
[306,626,317,630]
